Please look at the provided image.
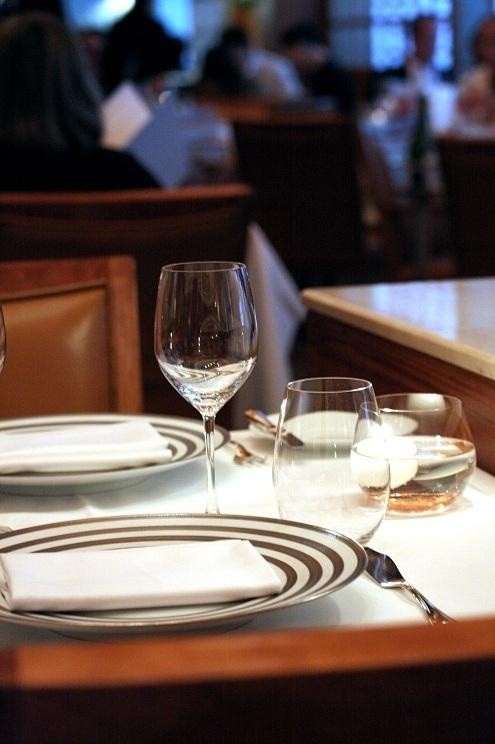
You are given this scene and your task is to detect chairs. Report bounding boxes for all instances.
[0,34,495,743]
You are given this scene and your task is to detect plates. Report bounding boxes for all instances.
[1,406,231,488]
[1,512,370,638]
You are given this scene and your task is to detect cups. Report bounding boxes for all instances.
[359,392,477,511]
[271,377,393,542]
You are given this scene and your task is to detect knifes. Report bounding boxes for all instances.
[246,407,304,450]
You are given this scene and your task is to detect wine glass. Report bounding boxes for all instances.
[149,259,258,518]
